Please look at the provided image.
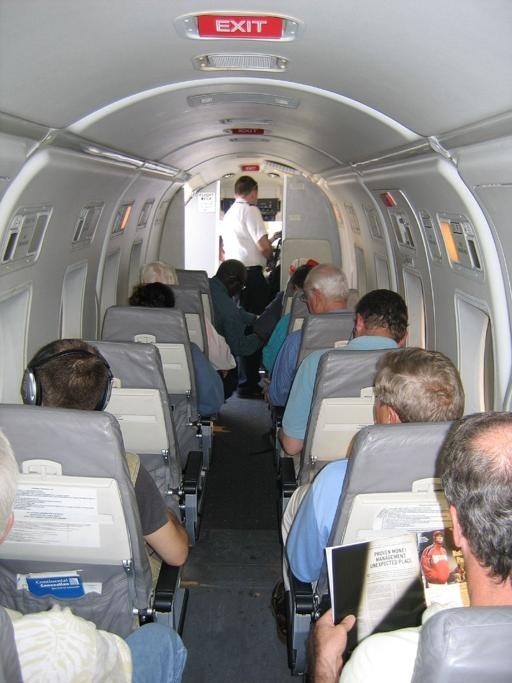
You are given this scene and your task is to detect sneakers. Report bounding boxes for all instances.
[270,578,288,642]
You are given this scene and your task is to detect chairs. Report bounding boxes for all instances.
[261,280,512,683]
[2,267,240,636]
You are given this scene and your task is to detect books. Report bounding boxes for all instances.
[324,518,475,666]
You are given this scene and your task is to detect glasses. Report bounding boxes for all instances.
[301,294,307,302]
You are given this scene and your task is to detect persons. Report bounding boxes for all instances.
[220,173,275,399]
[1,427,189,683]
[309,407,511,682]
[419,528,450,587]
[275,287,409,456]
[271,344,467,644]
[129,277,228,422]
[219,228,280,311]
[208,258,268,406]
[260,259,316,378]
[20,336,191,579]
[266,261,353,407]
[140,259,239,374]
